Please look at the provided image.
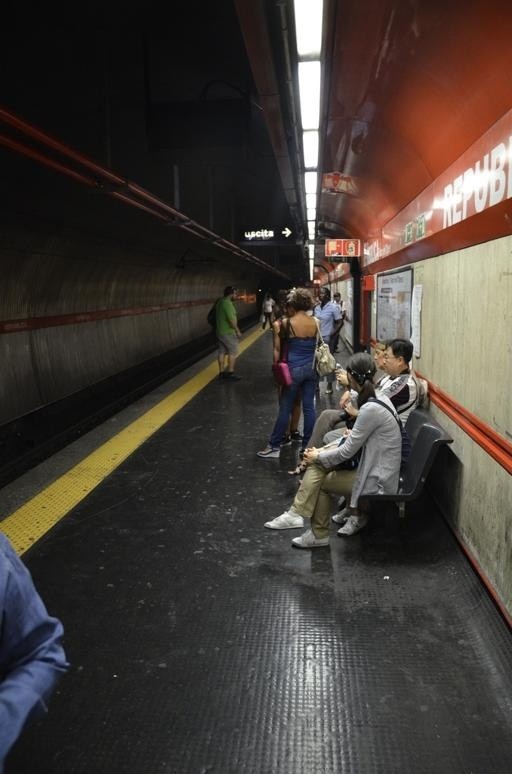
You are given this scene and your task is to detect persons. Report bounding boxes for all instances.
[259,285,345,458]
[1,528,68,771]
[211,286,243,382]
[265,339,417,548]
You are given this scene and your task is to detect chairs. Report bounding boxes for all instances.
[355,412,454,531]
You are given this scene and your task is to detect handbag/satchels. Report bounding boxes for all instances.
[207,297,222,327]
[272,362,292,386]
[315,343,336,378]
[334,437,358,471]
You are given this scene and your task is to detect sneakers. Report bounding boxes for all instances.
[264,511,305,530]
[280,429,303,447]
[325,388,333,394]
[218,372,243,381]
[331,507,368,537]
[256,444,281,458]
[290,528,329,549]
